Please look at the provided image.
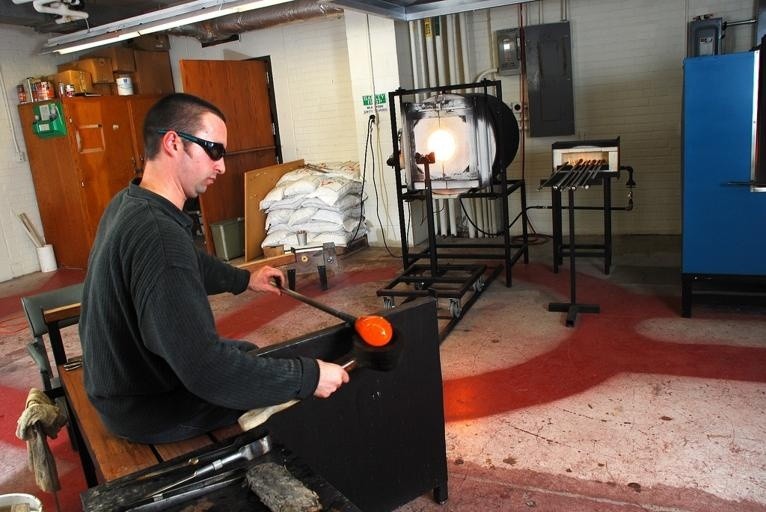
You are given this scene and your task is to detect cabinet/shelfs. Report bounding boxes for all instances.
[14,96,159,272]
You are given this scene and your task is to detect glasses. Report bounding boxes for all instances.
[156,130,226,160]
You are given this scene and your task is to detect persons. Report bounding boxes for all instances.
[79,93,350,447]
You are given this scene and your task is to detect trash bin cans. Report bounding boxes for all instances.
[209,216,244,261]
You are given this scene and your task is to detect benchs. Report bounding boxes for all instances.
[16,280,272,504]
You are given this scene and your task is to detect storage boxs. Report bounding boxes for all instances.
[51,30,176,102]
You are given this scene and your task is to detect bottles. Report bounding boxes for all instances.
[56,82,75,99]
[16,84,27,104]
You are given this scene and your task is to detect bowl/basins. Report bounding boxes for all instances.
[0,493,43,511]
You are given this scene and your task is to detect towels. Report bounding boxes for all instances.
[14,386,69,495]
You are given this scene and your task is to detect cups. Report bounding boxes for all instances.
[116,77,134,96]
